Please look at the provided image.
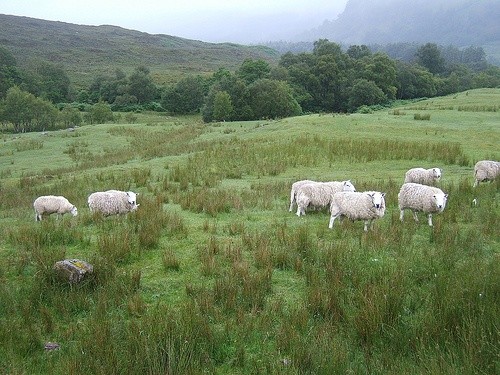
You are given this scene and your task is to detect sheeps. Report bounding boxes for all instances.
[33,195,77,222]
[289,179,357,218]
[404,167,441,185]
[329,191,386,231]
[473,160,500,188]
[398,183,448,227]
[88,190,140,217]
